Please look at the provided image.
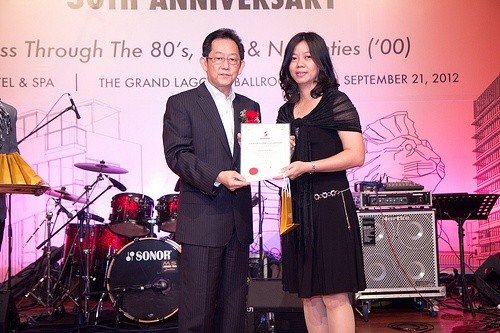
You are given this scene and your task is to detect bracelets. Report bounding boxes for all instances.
[310,161,315,173]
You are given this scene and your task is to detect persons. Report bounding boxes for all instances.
[163,28,261,333]
[273,32,367,333]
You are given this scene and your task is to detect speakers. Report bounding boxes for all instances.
[468,251,500,308]
[354,208,438,290]
[246,277,308,333]
[0,289,20,333]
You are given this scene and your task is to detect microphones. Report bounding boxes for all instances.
[141,279,167,289]
[52,198,73,218]
[105,175,127,191]
[88,213,105,222]
[68,94,80,119]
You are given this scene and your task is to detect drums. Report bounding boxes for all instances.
[61,224,94,274]
[95,224,131,273]
[109,191,153,237]
[106,238,182,324]
[156,192,181,233]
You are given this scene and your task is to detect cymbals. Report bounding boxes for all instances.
[43,187,94,205]
[74,162,130,174]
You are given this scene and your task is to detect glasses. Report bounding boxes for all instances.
[205,56,239,66]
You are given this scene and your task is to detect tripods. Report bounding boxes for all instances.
[15,172,106,325]
[431,193,500,317]
